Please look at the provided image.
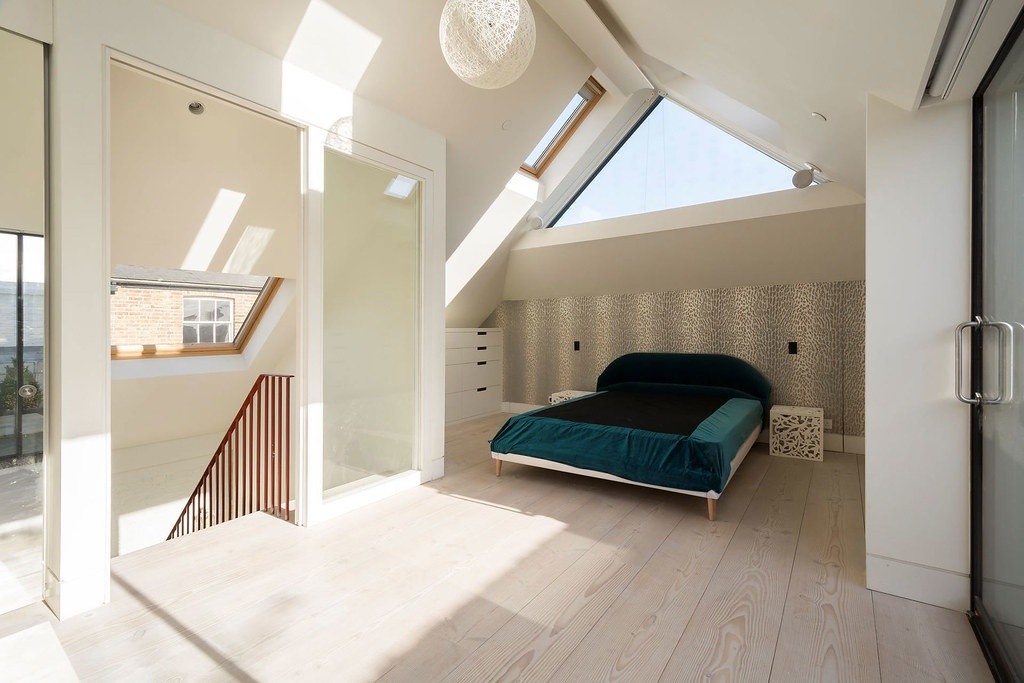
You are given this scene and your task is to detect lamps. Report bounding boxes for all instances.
[438,0,536,88]
[792,162,821,189]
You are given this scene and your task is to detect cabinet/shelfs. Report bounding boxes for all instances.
[444,328,503,428]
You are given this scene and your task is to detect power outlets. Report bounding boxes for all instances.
[824,419,832,429]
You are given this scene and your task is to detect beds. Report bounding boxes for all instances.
[489,352,773,522]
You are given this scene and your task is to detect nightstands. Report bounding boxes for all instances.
[551,390,596,406]
[768,404,825,461]
